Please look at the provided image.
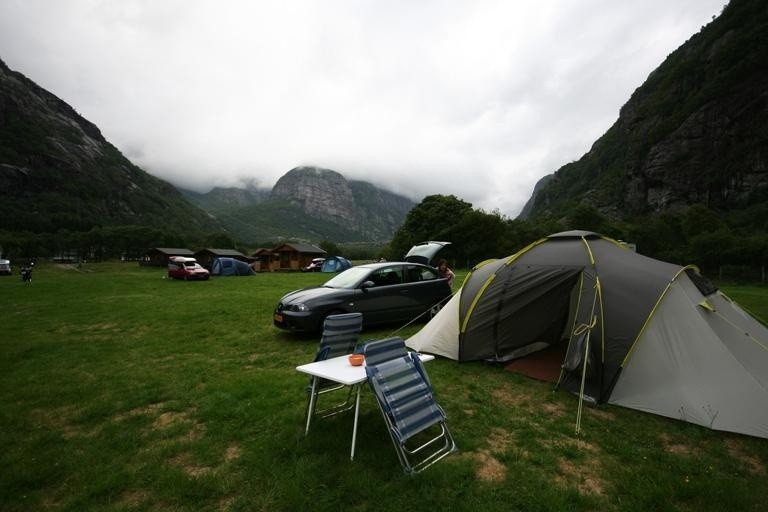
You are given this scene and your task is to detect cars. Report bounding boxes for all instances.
[168,256,210,280]
[300,257,326,272]
[0,264,12,275]
[272,241,453,342]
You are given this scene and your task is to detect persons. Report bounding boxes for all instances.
[373,257,385,263]
[435,259,455,290]
[24,262,34,287]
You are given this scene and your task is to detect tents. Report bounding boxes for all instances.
[403,229,768,439]
[211,257,256,276]
[321,256,352,272]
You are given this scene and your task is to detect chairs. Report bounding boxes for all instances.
[370,271,401,285]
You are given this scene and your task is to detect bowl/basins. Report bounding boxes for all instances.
[350,354,364,367]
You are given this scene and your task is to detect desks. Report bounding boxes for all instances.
[296,351,437,461]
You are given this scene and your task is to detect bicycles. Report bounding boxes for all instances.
[24,267,33,288]
